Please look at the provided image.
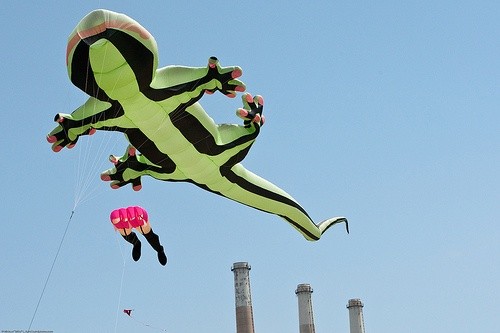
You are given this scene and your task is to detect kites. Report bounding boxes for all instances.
[110,206,167,266]
[46,9,349,242]
[124,309,154,329]
